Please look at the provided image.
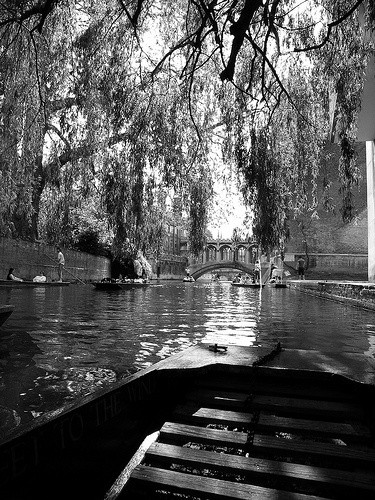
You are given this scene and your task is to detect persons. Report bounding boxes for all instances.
[232,273,241,283]
[32,271,46,282]
[296,255,307,280]
[217,273,220,279]
[57,248,65,282]
[271,264,282,283]
[185,267,190,279]
[8,267,23,281]
[255,260,261,283]
[142,269,147,284]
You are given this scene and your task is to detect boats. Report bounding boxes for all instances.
[231,280,266,288]
[0,279,70,287]
[183,277,195,282]
[268,282,286,288]
[90,280,154,290]
